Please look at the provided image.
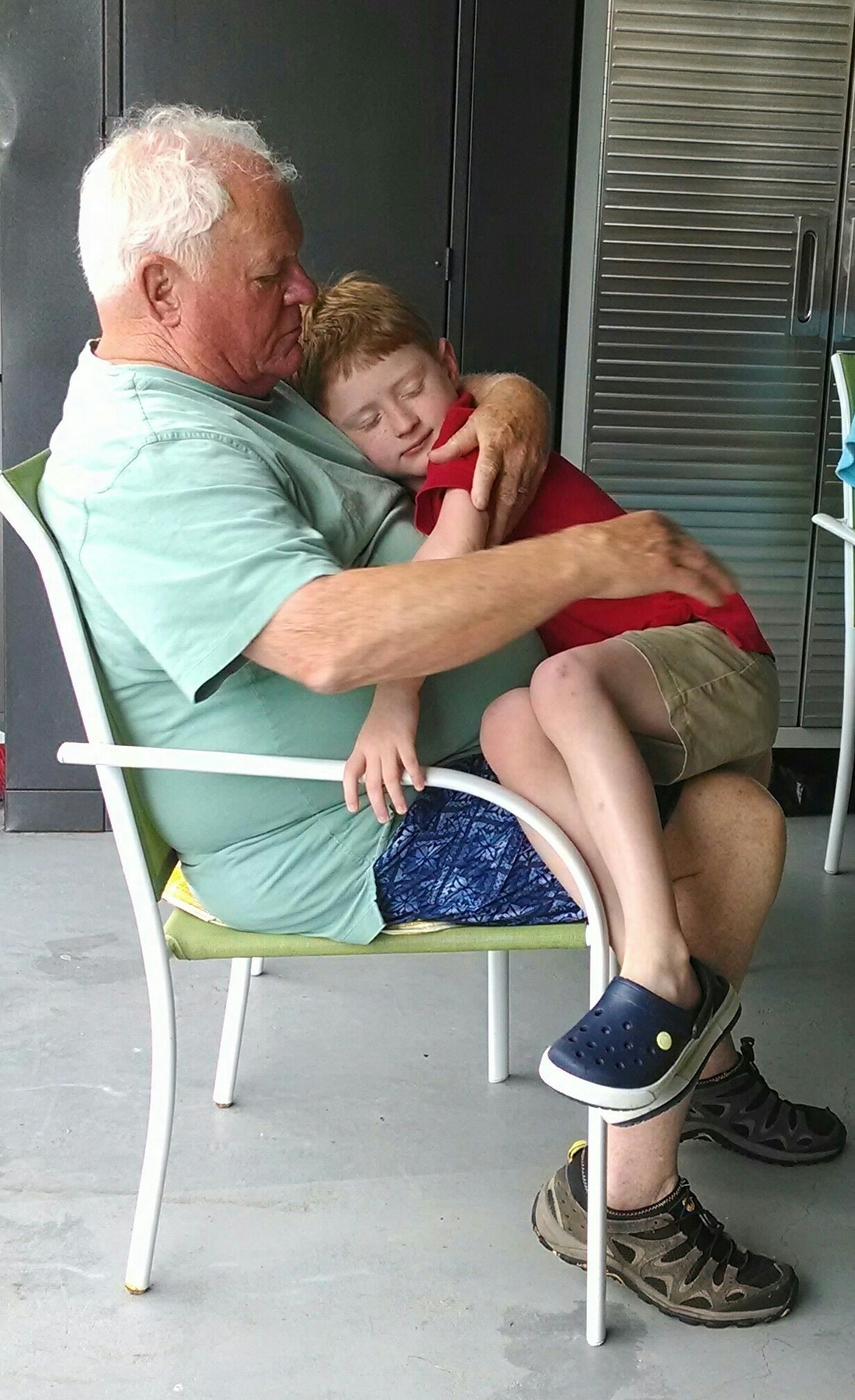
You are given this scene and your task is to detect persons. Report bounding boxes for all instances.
[292,268,784,1127]
[38,102,844,1329]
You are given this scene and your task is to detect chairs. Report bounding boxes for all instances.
[0,450,618,1348]
[811,352,854,877]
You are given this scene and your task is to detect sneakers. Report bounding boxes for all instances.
[678,1037,846,1167]
[531,1141,800,1329]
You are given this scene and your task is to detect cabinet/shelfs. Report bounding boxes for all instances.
[0,0,855,833]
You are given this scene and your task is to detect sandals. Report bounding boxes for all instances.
[539,956,741,1128]
